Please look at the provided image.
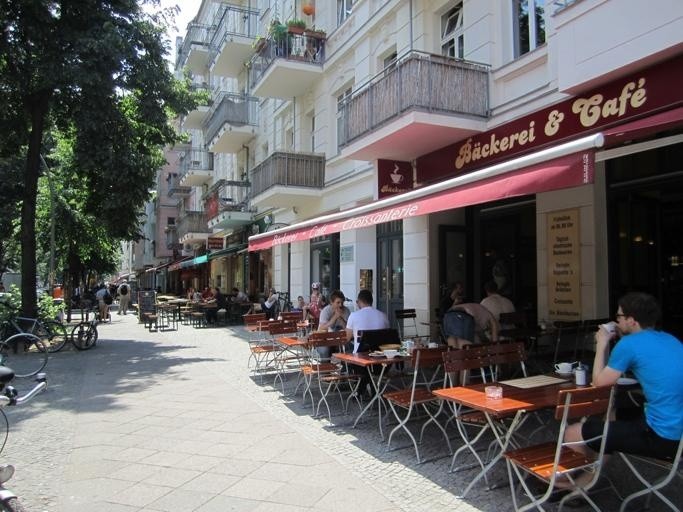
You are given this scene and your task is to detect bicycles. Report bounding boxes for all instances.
[72,300,98,351]
[0,300,67,352]
[0,332,48,378]
[0,363,47,512]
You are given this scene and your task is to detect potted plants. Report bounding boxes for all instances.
[251,20,328,61]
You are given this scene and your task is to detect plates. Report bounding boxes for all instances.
[616,377,637,385]
[369,352,386,357]
[554,369,570,374]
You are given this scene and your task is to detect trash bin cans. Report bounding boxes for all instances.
[53,298,64,321]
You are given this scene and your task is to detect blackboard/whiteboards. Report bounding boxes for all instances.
[138,291,156,322]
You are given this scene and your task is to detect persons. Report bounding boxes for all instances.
[77,279,131,323]
[316,288,390,398]
[157,286,163,294]
[443,266,516,384]
[188,282,329,331]
[565,292,683,460]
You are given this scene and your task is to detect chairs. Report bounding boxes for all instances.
[278,311,304,363]
[441,348,520,475]
[268,320,310,390]
[250,319,283,386]
[481,341,553,462]
[309,338,363,428]
[299,330,346,417]
[495,311,527,347]
[341,327,397,410]
[502,383,618,510]
[130,293,220,332]
[393,308,432,343]
[457,341,496,386]
[616,416,683,511]
[241,313,277,368]
[383,346,453,465]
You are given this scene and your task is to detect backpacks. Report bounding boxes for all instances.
[120,285,127,295]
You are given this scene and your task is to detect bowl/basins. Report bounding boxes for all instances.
[379,344,400,350]
[383,350,396,358]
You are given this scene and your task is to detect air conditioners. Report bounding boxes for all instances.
[183,242,193,252]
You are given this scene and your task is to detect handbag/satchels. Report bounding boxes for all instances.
[104,292,113,305]
[96,296,103,300]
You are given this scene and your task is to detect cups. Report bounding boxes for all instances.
[554,362,571,371]
[484,385,502,400]
[427,342,438,348]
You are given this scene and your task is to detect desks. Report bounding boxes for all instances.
[274,332,346,398]
[332,341,446,444]
[247,320,317,380]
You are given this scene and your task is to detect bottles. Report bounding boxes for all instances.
[575,361,585,385]
